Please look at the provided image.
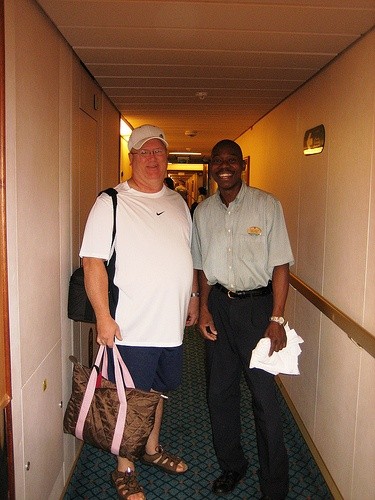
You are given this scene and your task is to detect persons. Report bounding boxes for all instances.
[78,124,199,500]
[163,176,209,219]
[192,140,295,500]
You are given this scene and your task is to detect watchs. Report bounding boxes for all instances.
[191,293,199,297]
[271,316,287,326]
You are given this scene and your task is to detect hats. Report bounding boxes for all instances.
[127,124,168,151]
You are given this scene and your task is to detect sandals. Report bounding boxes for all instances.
[140,449,189,474]
[110,469,147,500]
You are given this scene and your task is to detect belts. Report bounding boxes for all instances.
[214,282,272,299]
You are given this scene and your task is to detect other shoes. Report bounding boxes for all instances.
[213,466,247,494]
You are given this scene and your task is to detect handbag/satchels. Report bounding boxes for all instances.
[68,267,119,323]
[64,343,162,461]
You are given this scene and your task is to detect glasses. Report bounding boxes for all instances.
[133,149,167,157]
